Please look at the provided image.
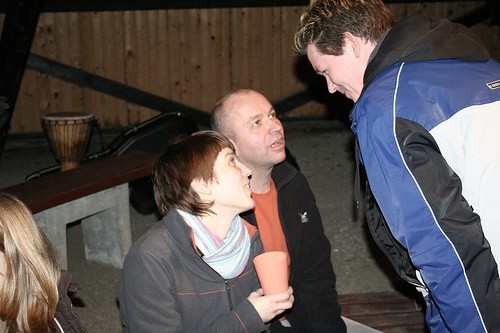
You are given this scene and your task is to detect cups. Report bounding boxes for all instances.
[253,251,288,295]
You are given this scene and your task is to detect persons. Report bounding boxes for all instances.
[211,88,347,333]
[0,193,87,333]
[293,0,500,332]
[117,130,294,333]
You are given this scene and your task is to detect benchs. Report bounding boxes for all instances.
[0,148,161,270]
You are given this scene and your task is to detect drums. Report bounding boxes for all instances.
[40,111,96,172]
